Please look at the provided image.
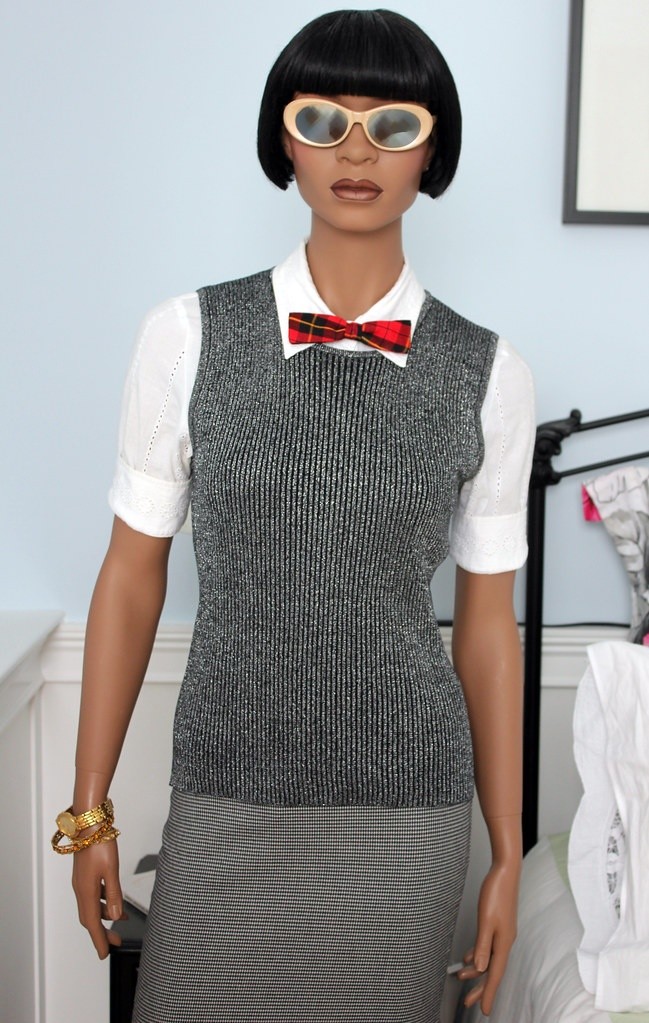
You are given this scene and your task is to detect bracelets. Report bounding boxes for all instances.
[72,827,120,848]
[51,814,115,855]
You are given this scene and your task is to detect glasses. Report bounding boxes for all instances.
[284,98,435,151]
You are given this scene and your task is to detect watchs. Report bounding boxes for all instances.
[56,797,114,840]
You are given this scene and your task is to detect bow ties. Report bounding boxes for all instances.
[289,312,414,355]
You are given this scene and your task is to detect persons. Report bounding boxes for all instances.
[51,9,538,1023]
